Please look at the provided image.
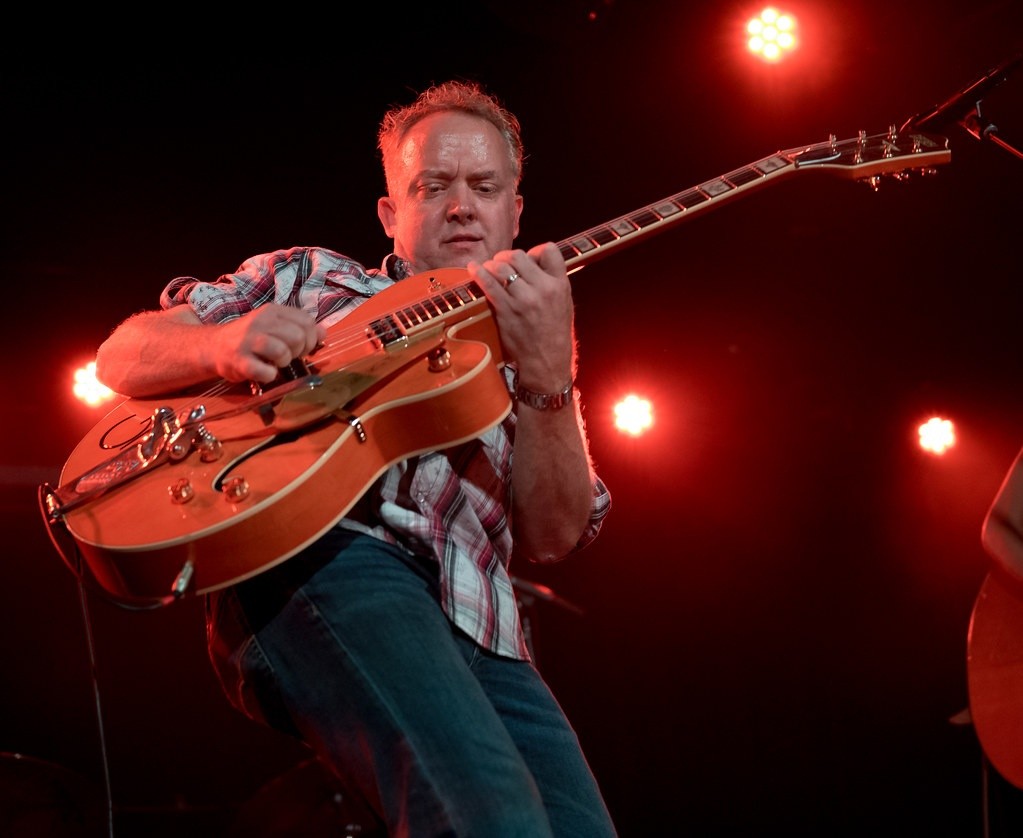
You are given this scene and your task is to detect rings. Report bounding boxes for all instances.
[504,273,520,291]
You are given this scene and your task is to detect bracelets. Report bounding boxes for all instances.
[513,369,574,413]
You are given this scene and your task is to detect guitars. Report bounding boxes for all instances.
[967,564,1023,792]
[53,127,956,613]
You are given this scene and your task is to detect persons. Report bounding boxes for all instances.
[981,446,1023,838]
[97,81,619,838]
